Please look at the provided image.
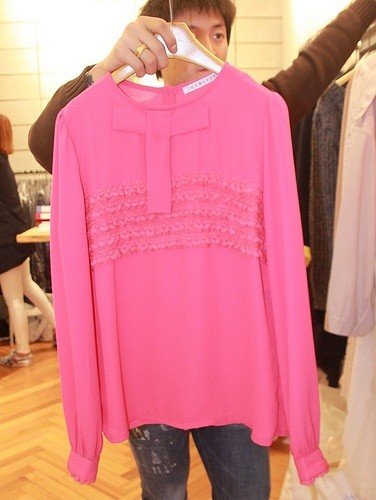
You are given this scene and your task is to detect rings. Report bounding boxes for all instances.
[134,44,148,59]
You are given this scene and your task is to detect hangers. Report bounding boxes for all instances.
[337,27,376,86]
[111,0,224,85]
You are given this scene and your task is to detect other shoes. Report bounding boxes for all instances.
[0,350,33,367]
[52,327,57,349]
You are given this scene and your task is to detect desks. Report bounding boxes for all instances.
[17,221,51,295]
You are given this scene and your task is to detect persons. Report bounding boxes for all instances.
[0,115,57,367]
[28,0,376,498]
